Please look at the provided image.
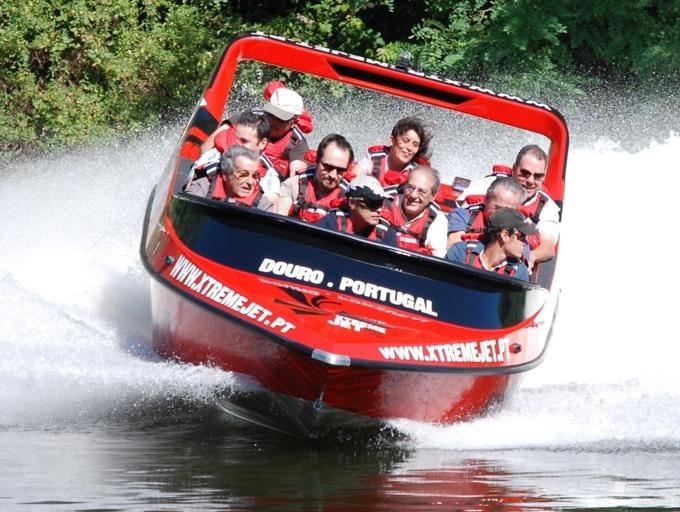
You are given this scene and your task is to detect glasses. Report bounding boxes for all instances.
[320,160,346,173]
[520,168,544,181]
[358,198,383,208]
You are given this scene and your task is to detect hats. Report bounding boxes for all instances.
[346,175,395,201]
[487,207,535,234]
[263,87,303,121]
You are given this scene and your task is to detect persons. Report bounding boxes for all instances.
[281,132,357,226]
[352,117,431,198]
[446,175,531,264]
[183,110,282,214]
[455,142,561,276]
[186,144,275,215]
[310,175,396,249]
[202,86,311,185]
[443,206,531,284]
[365,162,448,259]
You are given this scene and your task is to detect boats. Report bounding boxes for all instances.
[145,372,560,511]
[140,30,570,427]
